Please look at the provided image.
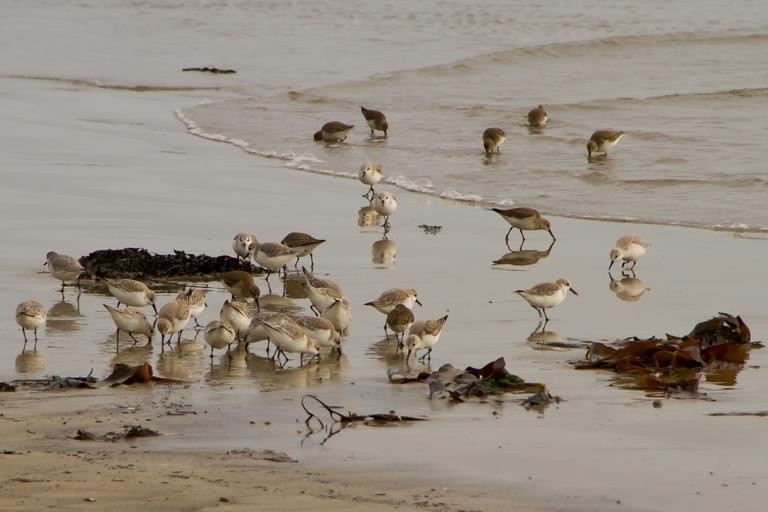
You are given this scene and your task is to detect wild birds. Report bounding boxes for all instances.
[363,288,423,329]
[371,191,398,227]
[585,129,624,158]
[14,299,47,342]
[313,120,354,143]
[512,278,579,321]
[487,242,555,271]
[360,106,388,137]
[527,104,548,127]
[483,206,557,241]
[405,315,448,361]
[384,302,416,346]
[608,234,650,270]
[100,231,350,369]
[482,127,507,154]
[607,271,652,303]
[43,250,90,287]
[358,163,383,196]
